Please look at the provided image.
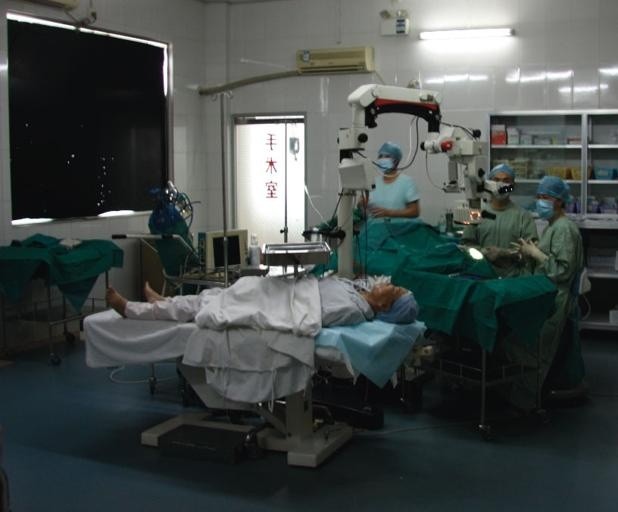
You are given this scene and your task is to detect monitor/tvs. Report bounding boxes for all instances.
[198,230,249,274]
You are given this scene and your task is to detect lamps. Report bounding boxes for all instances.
[419,28,515,40]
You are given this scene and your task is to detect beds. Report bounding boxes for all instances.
[83,308,426,469]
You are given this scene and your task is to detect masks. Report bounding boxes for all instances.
[375,158,396,175]
[535,199,556,221]
[492,182,512,201]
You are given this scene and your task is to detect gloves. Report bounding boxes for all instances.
[481,237,549,264]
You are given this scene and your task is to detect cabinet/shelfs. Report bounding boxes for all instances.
[486,110,618,334]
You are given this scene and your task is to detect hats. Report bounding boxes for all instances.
[536,175,573,205]
[378,142,402,161]
[488,163,516,179]
[376,291,420,324]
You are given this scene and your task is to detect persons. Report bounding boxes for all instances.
[458,163,540,282]
[504,173,586,372]
[104,274,421,331]
[354,140,421,224]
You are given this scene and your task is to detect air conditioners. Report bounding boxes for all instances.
[296,47,376,75]
